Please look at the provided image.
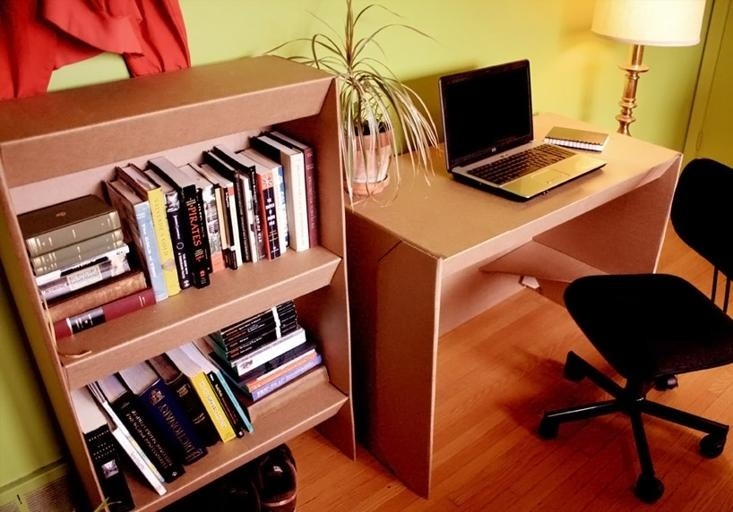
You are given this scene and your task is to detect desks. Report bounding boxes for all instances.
[338,110,684,497]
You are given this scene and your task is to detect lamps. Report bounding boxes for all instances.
[586,0,707,143]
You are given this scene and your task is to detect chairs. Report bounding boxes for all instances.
[536,157,732,506]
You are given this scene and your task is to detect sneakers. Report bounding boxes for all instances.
[222,443,299,511]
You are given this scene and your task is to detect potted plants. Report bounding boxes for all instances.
[243,1,445,211]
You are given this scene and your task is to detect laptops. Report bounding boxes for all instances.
[438,60,608,199]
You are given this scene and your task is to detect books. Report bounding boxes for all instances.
[543,126,609,152]
[69,300,331,511]
[18,130,319,340]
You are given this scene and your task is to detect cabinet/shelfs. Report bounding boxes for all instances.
[0,56,359,512]
